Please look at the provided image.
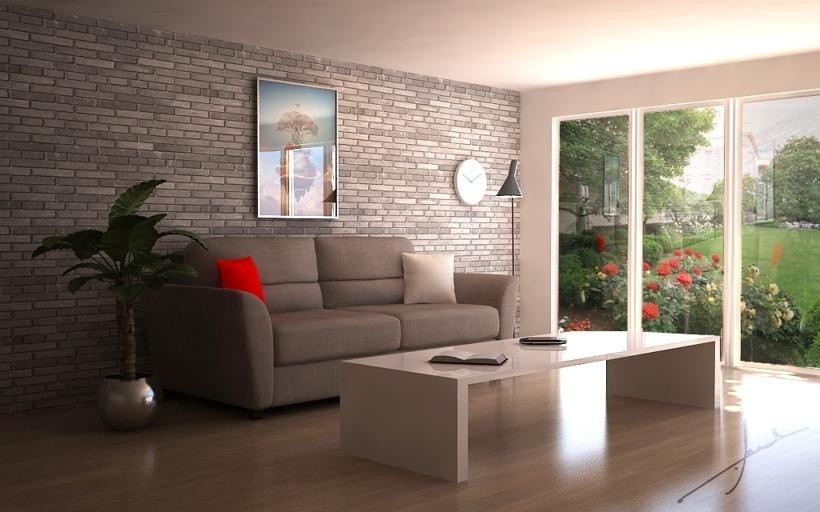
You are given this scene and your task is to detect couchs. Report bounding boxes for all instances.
[138,235,519,417]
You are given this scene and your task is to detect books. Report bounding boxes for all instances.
[518,334,567,346]
[427,349,508,366]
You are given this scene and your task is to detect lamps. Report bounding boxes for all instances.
[496,158,523,338]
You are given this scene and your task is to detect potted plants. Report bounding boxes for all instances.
[31,179,209,434]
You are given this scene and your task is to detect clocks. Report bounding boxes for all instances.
[453,158,489,206]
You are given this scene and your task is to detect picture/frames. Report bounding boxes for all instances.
[256,76,341,222]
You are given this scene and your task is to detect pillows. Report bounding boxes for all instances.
[401,252,456,305]
[214,257,266,306]
